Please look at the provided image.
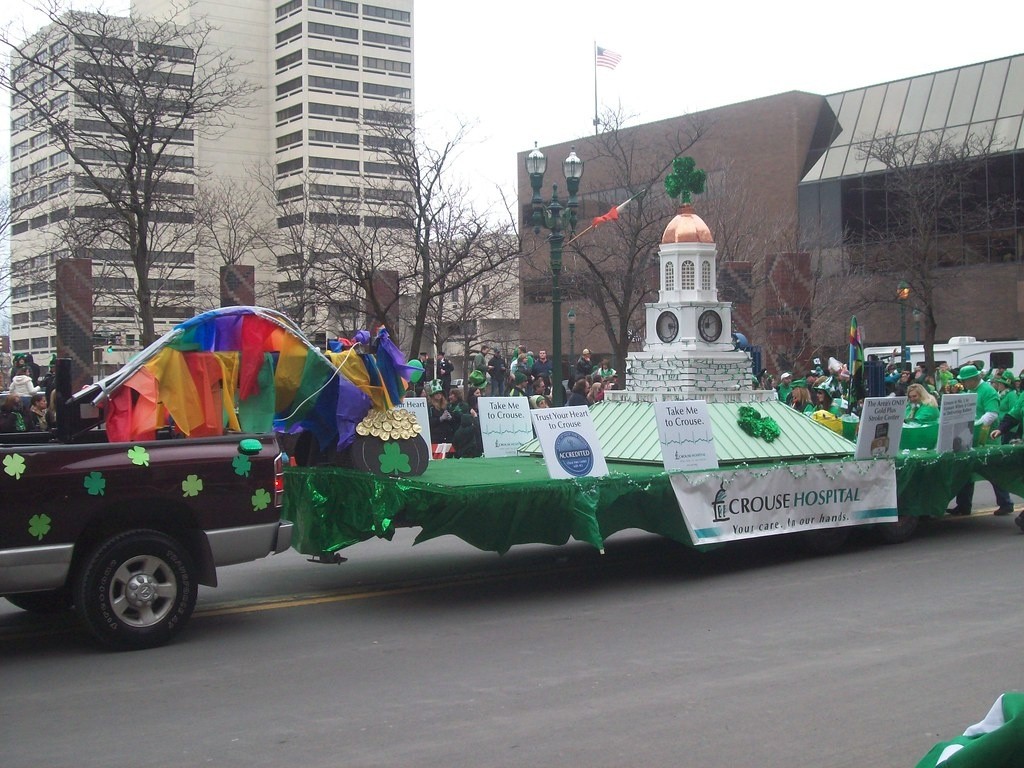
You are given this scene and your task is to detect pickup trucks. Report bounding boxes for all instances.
[0,432,296,654]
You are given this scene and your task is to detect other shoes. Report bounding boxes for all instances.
[945,505,971,515]
[993,505,1015,515]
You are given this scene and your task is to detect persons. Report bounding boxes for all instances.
[416,345,619,458]
[754,348,1024,528]
[0,353,59,443]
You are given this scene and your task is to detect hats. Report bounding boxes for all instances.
[536,395,546,403]
[592,365,599,370]
[514,372,528,385]
[468,370,488,390]
[582,349,591,355]
[494,347,501,353]
[814,382,833,399]
[47,354,56,367]
[815,366,823,373]
[428,379,445,396]
[789,380,809,388]
[828,356,843,374]
[949,379,958,387]
[438,351,445,355]
[987,363,1024,388]
[956,365,982,380]
[420,352,428,356]
[780,372,791,380]
[516,353,526,362]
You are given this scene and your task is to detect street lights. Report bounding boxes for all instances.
[567,307,576,364]
[526,141,584,405]
[896,280,910,372]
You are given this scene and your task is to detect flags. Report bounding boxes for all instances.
[592,199,632,225]
[97,312,423,451]
[596,46,623,71]
[850,315,865,377]
